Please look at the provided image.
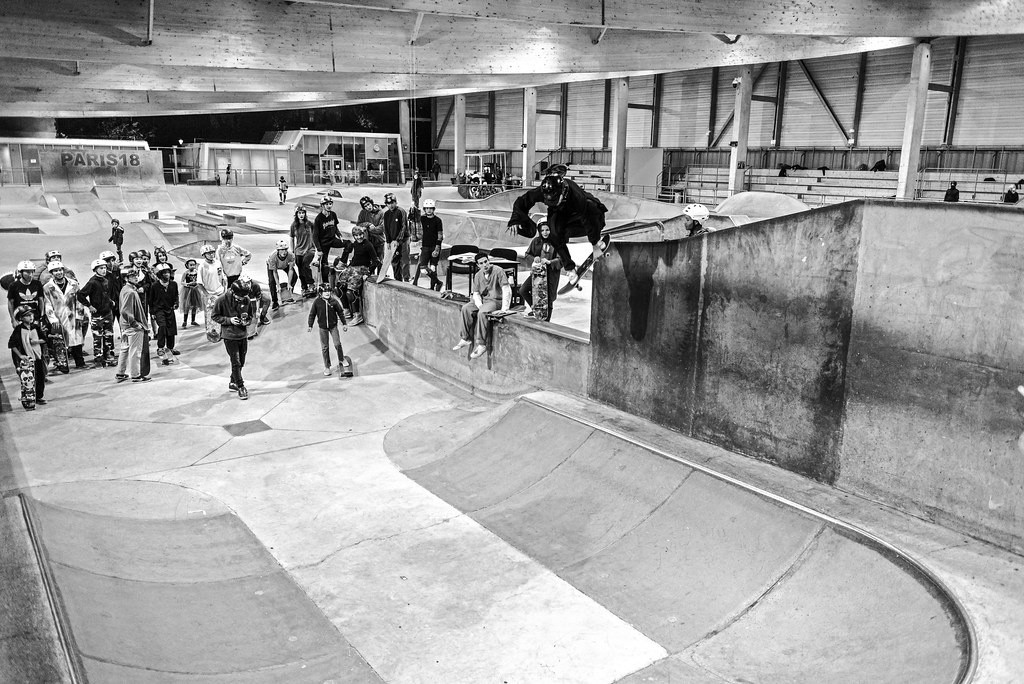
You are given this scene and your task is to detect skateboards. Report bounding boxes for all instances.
[157,348,175,365]
[557,234,611,295]
[408,247,422,285]
[279,201,285,205]
[656,220,669,241]
[339,356,354,378]
[140,331,151,377]
[311,256,321,294]
[532,259,549,322]
[376,244,399,283]
[246,313,259,339]
[16,356,36,410]
[485,305,527,323]
[279,282,296,303]
[48,324,69,374]
[203,294,222,342]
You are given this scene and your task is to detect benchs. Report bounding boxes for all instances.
[685,166,1024,204]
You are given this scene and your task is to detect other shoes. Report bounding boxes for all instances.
[229,383,239,391]
[261,285,314,318]
[338,312,352,320]
[525,312,534,318]
[36,347,180,405]
[348,317,363,325]
[181,322,200,328]
[435,281,443,291]
[238,387,248,399]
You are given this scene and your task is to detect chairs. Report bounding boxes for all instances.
[445,244,479,297]
[489,248,517,306]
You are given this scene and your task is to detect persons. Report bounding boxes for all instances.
[453,253,512,358]
[683,204,709,238]
[33,228,272,400]
[519,217,564,322]
[226,163,231,185]
[1004,184,1019,203]
[943,181,960,203]
[278,176,288,203]
[267,195,443,376]
[7,260,49,384]
[7,305,47,409]
[108,219,124,265]
[485,169,492,184]
[470,171,479,195]
[495,166,513,191]
[504,174,606,285]
[431,160,442,181]
[411,172,423,208]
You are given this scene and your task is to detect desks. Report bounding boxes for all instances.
[448,259,521,304]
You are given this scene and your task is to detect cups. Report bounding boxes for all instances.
[241,313,248,325]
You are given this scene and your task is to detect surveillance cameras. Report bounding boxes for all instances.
[732,81,737,88]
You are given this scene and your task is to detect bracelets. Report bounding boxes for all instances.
[40,316,44,322]
[436,240,441,245]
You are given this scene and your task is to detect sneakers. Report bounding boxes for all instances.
[566,267,578,285]
[470,346,486,358]
[324,367,331,376]
[595,240,606,249]
[340,358,349,366]
[453,339,472,350]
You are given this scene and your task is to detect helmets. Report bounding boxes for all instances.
[200,228,233,256]
[185,259,196,268]
[16,247,170,276]
[292,192,436,234]
[537,217,548,228]
[13,305,35,320]
[541,177,561,200]
[319,282,331,294]
[112,219,119,224]
[238,275,251,283]
[231,280,250,297]
[276,240,288,250]
